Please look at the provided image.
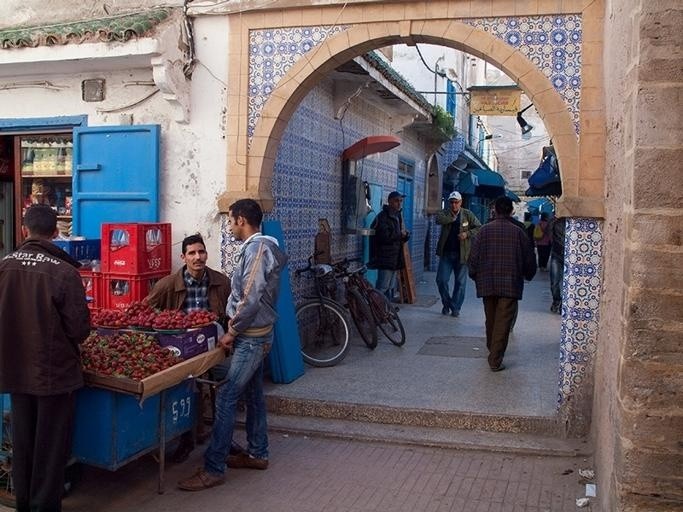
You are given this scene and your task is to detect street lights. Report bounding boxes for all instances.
[475,133,501,155]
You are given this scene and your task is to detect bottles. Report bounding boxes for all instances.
[63,188,72,216]
[76,227,162,301]
[20,140,71,176]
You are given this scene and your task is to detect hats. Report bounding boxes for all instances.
[447,191,462,201]
[388,191,407,200]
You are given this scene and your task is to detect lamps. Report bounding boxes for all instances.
[516,102,536,135]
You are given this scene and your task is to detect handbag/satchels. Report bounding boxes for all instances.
[533,224,544,238]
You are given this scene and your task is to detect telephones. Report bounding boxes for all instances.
[348,174,372,219]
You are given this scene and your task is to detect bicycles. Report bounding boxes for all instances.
[337,267,405,348]
[290,255,377,368]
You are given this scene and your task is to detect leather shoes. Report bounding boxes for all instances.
[178,470,225,490]
[227,452,268,470]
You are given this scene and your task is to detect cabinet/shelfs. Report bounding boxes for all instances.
[15,135,73,248]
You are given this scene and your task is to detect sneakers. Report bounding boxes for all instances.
[442,304,460,317]
[493,363,506,370]
[550,299,561,313]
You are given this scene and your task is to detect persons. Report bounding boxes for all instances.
[434,191,481,316]
[369,192,411,297]
[534,213,552,272]
[549,211,566,317]
[510,207,526,230]
[468,197,536,373]
[145,233,230,322]
[0,203,92,511]
[487,200,498,221]
[179,199,288,490]
[521,212,533,242]
